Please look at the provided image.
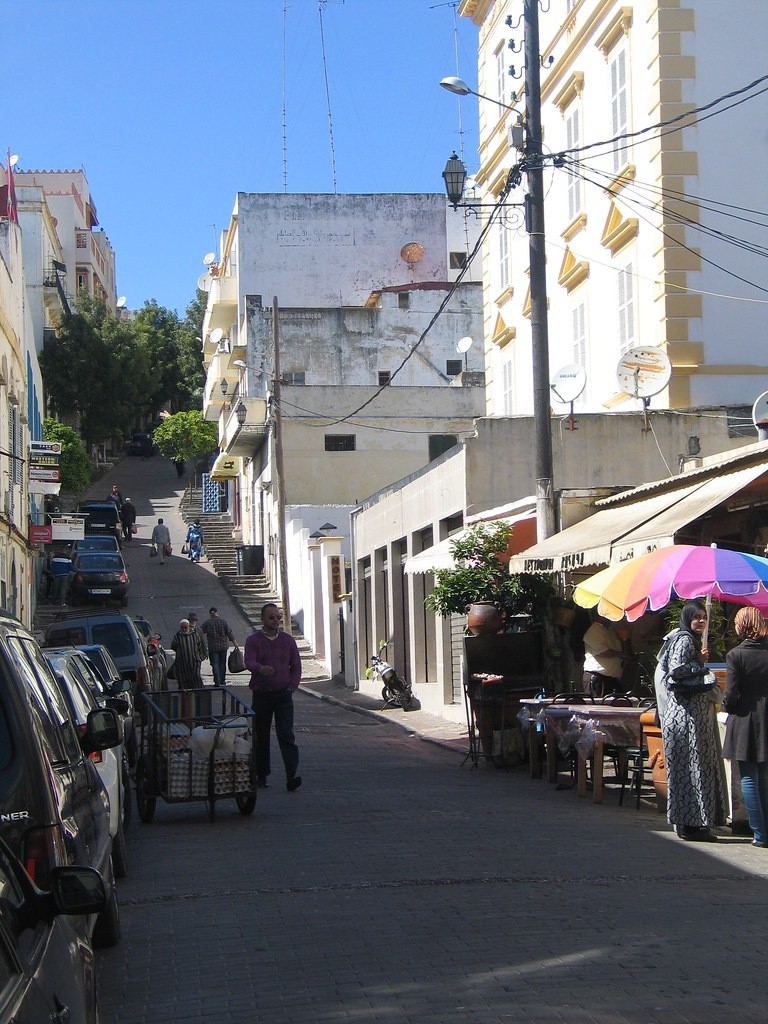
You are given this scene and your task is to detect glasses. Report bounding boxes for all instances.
[264,615,283,621]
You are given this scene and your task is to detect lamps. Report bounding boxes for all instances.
[320,523,337,537]
[309,531,326,545]
[220,378,247,398]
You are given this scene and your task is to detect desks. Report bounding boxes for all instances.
[544,704,612,783]
[519,696,647,779]
[568,707,656,797]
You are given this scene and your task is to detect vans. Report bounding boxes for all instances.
[44,608,153,725]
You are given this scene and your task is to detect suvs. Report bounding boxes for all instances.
[130,613,168,690]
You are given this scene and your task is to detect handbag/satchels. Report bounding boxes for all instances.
[228,649,247,674]
[666,634,717,692]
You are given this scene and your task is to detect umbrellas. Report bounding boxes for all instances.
[573,543,768,648]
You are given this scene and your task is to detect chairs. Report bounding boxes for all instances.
[533,690,661,810]
[107,560,116,568]
[103,541,111,550]
[83,559,91,568]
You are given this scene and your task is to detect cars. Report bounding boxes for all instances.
[1,607,123,951]
[67,551,130,608]
[49,644,137,825]
[67,536,126,564]
[70,500,124,549]
[0,836,111,1024]
[43,652,127,880]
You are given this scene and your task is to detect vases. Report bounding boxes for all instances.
[510,614,532,633]
[466,600,503,636]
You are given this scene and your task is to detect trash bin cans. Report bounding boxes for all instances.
[234,545,264,575]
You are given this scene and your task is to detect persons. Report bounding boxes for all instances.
[172,457,185,477]
[171,607,238,690]
[721,607,768,846]
[121,498,136,541]
[662,603,730,842]
[186,519,204,563]
[245,604,302,790]
[111,486,123,511]
[152,518,170,564]
[44,546,81,606]
[45,494,62,524]
[584,610,629,698]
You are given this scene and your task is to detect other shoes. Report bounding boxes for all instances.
[287,776,302,791]
[751,837,768,848]
[677,824,717,842]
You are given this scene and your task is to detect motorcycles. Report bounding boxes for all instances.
[365,640,413,712]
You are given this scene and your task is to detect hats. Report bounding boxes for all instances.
[180,618,189,624]
[210,607,218,615]
[187,613,199,622]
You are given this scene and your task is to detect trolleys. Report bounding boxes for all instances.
[136,686,257,821]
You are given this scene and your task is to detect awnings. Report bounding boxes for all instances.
[404,516,537,574]
[509,463,768,574]
[210,453,239,481]
